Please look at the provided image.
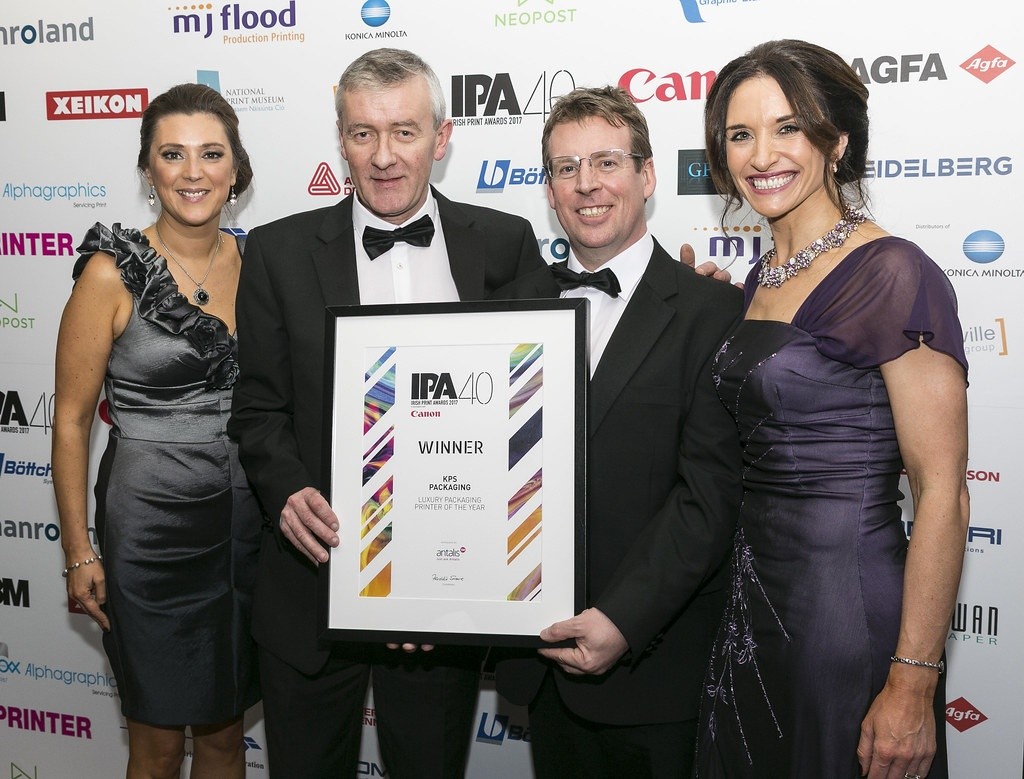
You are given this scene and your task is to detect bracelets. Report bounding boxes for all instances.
[61,555,103,579]
[891,655,945,673]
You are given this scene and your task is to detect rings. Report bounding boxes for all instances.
[905,773,920,779]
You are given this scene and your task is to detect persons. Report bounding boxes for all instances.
[704,39,971,779]
[233,45,743,779]
[485,86,745,779]
[53,84,266,779]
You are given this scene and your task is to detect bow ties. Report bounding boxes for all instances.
[551,262,621,298]
[363,214,435,261]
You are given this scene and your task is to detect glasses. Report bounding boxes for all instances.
[547,148,644,178]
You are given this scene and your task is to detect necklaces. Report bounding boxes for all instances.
[154,221,225,306]
[755,209,867,286]
